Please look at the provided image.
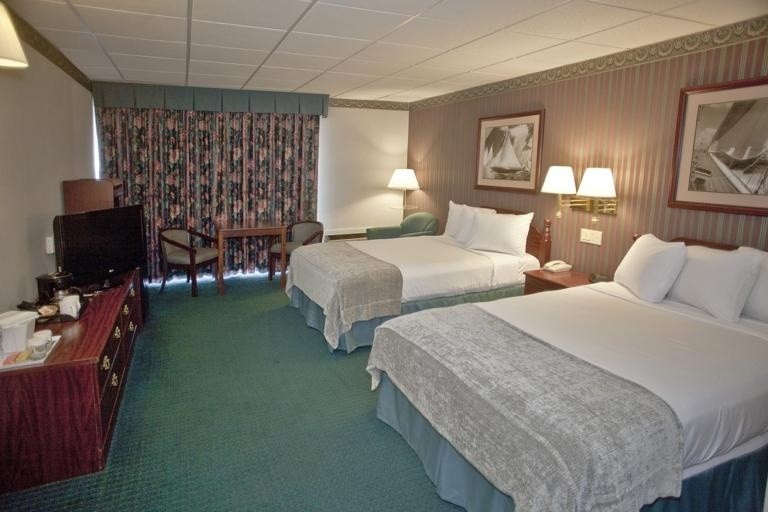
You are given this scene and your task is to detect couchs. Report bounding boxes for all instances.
[365,211,438,238]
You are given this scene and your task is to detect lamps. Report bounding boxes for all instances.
[387,169,420,209]
[0,2,31,71]
[541,166,577,210]
[577,170,617,215]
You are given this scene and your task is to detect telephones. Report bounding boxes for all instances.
[544,260,572,272]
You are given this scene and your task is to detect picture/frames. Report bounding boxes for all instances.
[666,77,768,216]
[472,108,547,196]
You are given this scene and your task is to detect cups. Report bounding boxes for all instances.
[29,338,48,360]
[58,300,78,319]
[35,330,52,347]
[63,295,81,316]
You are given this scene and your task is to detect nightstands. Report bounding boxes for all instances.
[523,267,610,296]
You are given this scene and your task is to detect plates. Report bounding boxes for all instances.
[1,336,62,368]
[38,298,89,322]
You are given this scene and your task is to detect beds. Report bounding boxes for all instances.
[368,240,768,512]
[287,205,552,351]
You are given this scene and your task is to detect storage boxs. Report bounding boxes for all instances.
[2,309,40,355]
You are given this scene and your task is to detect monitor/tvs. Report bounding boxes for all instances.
[52,203,149,295]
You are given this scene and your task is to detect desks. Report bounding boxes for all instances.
[0,265,144,495]
[217,222,287,294]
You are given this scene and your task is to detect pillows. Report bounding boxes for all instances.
[466,211,535,256]
[444,200,497,248]
[667,245,764,325]
[613,234,686,304]
[744,255,768,326]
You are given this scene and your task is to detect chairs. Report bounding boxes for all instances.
[158,227,226,298]
[269,221,324,280]
